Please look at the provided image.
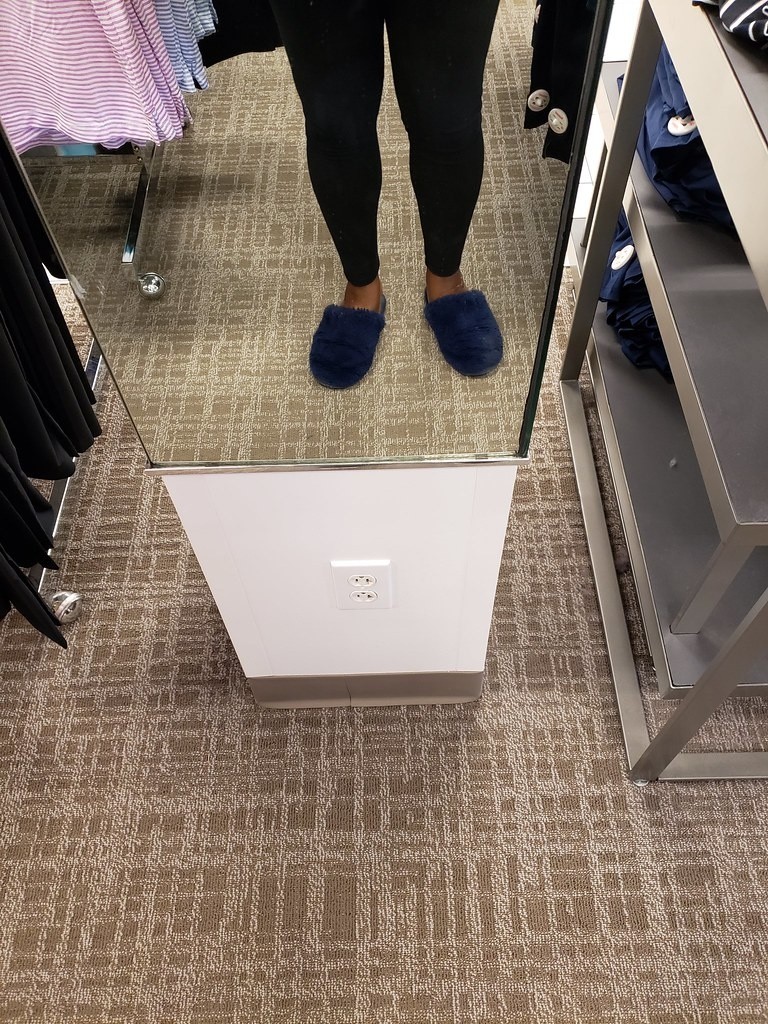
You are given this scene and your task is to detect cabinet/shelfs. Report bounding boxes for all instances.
[558,0,768,788]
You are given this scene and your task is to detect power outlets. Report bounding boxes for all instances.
[331,559,392,610]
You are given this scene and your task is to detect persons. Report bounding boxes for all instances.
[279,0,506,387]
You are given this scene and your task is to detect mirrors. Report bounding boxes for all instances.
[0,0,611,470]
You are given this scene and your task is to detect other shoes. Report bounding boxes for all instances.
[309,295,387,390]
[424,285,503,380]
